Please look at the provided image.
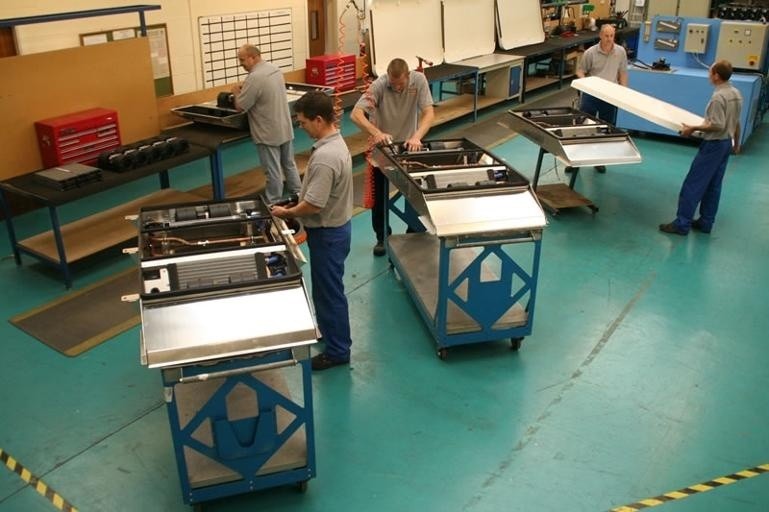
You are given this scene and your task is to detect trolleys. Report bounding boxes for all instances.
[531,146,601,218]
[159,344,319,511]
[383,175,544,361]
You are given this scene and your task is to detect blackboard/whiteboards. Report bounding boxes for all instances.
[365,0,546,77]
[0,38,163,181]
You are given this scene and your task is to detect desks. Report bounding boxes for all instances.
[0,24,643,290]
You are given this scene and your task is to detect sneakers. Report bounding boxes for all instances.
[692,218,711,233]
[659,222,679,234]
[373,240,387,257]
[310,348,348,370]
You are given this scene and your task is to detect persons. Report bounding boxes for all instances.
[231,43,302,206]
[564,23,628,174]
[271,91,354,371]
[658,59,743,236]
[349,58,435,256]
